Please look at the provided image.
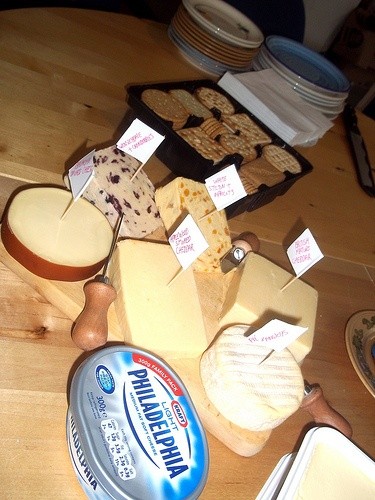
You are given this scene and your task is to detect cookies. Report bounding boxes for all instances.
[142,87,302,194]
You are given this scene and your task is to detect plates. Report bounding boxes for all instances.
[344,310,375,397]
[167,0,264,77]
[252,35,352,121]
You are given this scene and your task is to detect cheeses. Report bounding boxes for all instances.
[219,251,319,365]
[67,346,210,500]
[255,426,375,500]
[200,324,305,432]
[1,186,115,282]
[63,145,163,244]
[105,240,208,359]
[154,176,232,272]
